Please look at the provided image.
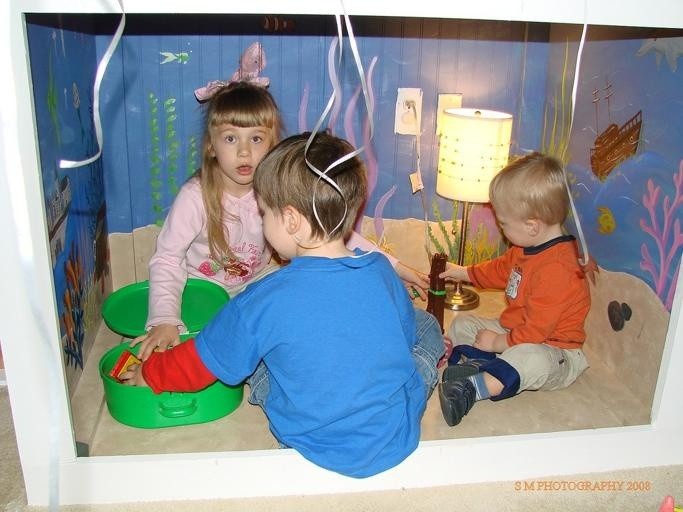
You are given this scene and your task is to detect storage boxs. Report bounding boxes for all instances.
[98,277,244,428]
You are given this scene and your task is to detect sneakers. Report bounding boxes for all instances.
[443,359,487,383]
[438,377,476,427]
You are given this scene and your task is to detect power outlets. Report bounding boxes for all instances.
[394,88,423,136]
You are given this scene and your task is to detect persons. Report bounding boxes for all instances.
[118,131,446,478]
[128,42,434,364]
[432,152,589,428]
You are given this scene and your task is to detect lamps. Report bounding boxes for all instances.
[436,108,513,312]
